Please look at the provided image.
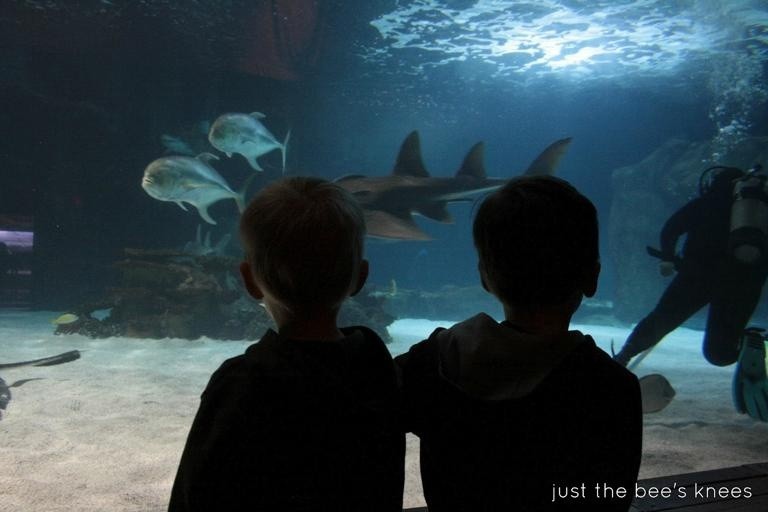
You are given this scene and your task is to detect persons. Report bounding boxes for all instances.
[165,177,404,509]
[614,164,766,371]
[389,172,642,510]
